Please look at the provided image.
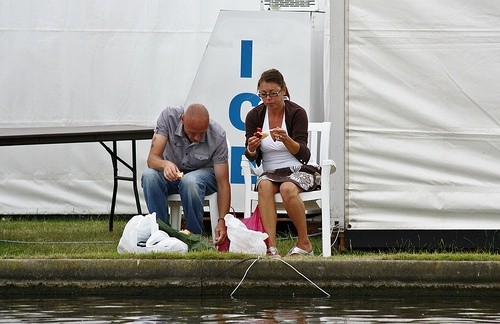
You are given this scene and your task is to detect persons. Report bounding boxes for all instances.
[244,69,321,260]
[141,104,232,246]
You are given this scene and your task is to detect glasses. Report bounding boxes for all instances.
[258,87,283,97]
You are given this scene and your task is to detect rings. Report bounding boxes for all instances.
[280,135,282,139]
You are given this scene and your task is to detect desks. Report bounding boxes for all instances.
[0,125,155,236]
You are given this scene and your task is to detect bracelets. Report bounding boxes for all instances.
[217,218,225,222]
[247,145,256,154]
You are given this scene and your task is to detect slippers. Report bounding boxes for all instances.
[267,247,279,257]
[286,247,314,257]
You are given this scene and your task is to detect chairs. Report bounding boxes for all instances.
[241,121,337,257]
[167,139,231,251]
